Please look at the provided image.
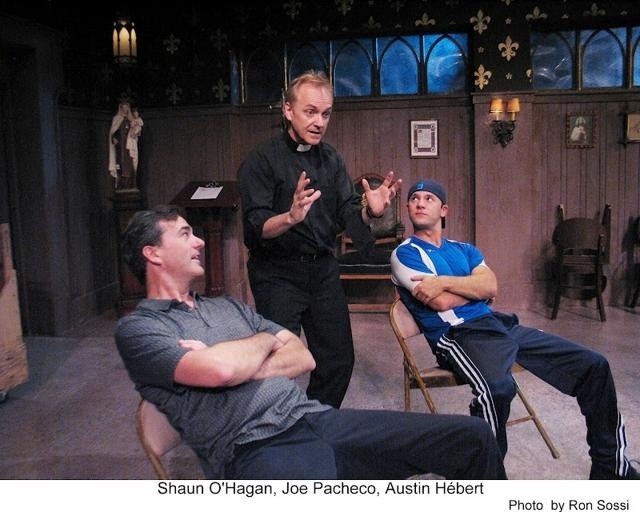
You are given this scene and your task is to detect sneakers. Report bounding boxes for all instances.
[590,460,640,480]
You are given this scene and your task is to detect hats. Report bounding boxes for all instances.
[409,179,445,230]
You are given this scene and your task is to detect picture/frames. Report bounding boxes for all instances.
[566,111,640,150]
[409,119,439,159]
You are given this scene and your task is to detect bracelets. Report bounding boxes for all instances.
[365,204,385,220]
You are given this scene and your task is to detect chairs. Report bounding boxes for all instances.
[137,172,640,479]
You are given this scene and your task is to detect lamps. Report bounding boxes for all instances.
[488,97,520,147]
[111,0,138,67]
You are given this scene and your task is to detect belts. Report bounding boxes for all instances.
[248,250,331,263]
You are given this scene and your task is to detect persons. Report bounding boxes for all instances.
[235,71,400,413]
[391,178,640,480]
[109,101,140,193]
[128,109,144,138]
[112,208,511,480]
